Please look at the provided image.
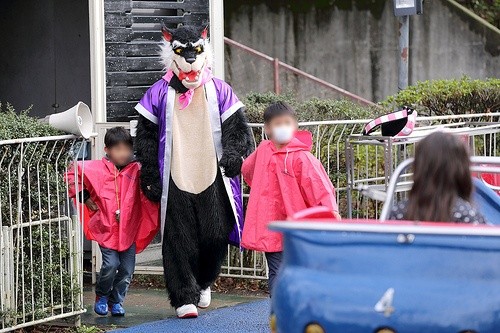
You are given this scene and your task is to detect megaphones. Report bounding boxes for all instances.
[36,101,93,139]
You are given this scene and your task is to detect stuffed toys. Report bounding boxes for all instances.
[134,22,251,318]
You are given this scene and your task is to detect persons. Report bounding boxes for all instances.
[389,132,485,223]
[63,127,159,317]
[240,104,341,298]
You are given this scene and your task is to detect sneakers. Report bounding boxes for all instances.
[198,286,211,308]
[109,302,125,315]
[176,304,198,317]
[94,293,110,314]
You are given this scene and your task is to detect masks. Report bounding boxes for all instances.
[268,125,295,142]
